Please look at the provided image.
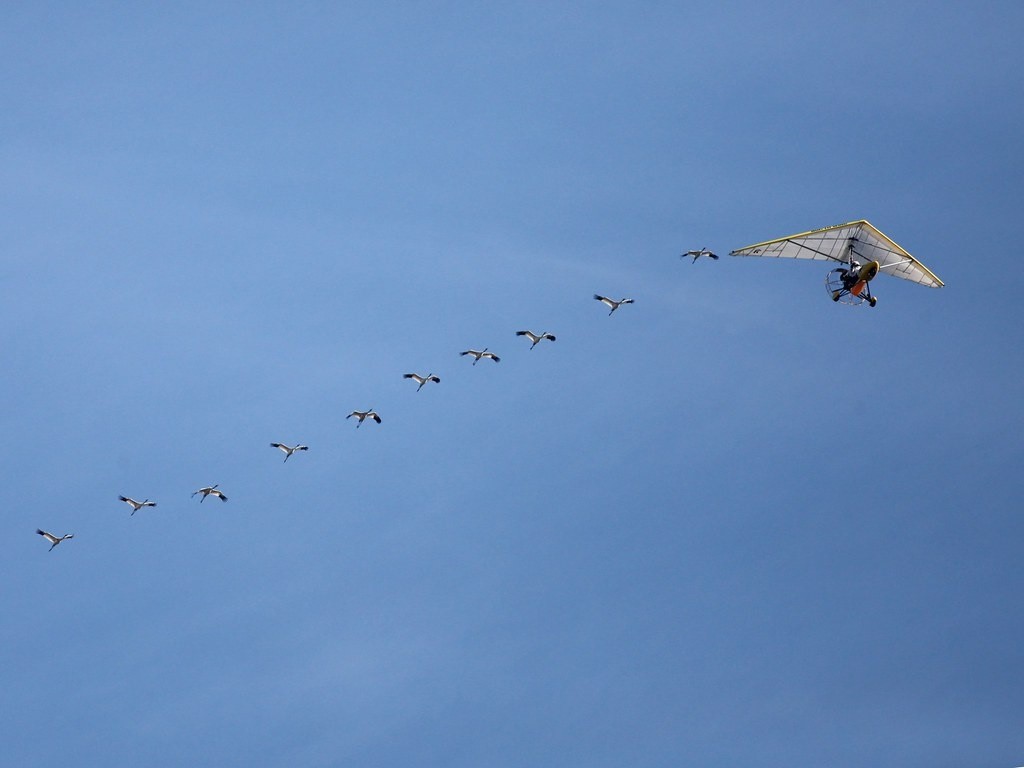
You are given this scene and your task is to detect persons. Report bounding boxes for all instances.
[852,261,861,271]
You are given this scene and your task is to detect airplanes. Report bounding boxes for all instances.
[728,220,944,307]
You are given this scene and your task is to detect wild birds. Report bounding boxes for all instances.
[594,293,635,316]
[35,528,75,553]
[269,442,309,464]
[516,330,557,351]
[346,408,382,429]
[117,493,158,516]
[191,484,229,504]
[459,348,501,366]
[681,246,720,265]
[402,373,440,393]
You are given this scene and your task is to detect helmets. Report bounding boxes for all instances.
[851,261,860,266]
